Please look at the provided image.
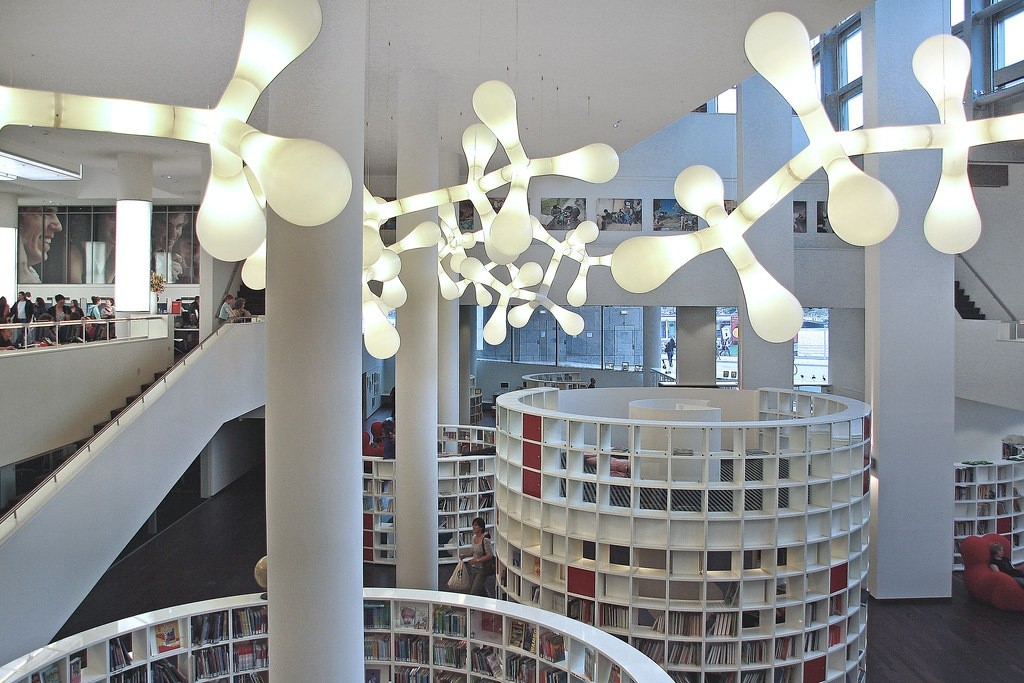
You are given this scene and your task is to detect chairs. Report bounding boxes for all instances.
[961,533,1024,612]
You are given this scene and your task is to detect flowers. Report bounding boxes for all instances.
[150,271,168,292]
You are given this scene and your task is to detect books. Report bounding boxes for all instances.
[954,467,1021,553]
[364,549,840,683]
[30,604,268,683]
[438,426,494,549]
[523,371,588,390]
[363,477,395,512]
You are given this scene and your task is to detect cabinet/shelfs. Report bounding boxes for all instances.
[0,372,1024,683]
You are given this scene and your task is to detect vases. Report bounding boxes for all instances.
[149,291,157,315]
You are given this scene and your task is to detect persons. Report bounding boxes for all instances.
[0,291,115,354]
[218,295,251,326]
[664,339,676,367]
[17,206,115,284]
[550,204,581,225]
[601,201,642,230]
[458,517,494,597]
[151,206,199,284]
[989,542,1024,588]
[588,377,597,388]
[173,296,202,329]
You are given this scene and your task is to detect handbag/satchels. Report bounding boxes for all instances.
[447,560,471,590]
[101,314,115,324]
[86,306,98,327]
[481,537,496,575]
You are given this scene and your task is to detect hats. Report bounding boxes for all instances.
[55,294,66,303]
[69,312,81,320]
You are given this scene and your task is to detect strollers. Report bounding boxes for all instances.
[557,212,568,225]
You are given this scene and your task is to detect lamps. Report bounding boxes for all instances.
[0,0,1024,361]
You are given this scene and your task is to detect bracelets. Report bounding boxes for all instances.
[477,558,480,563]
